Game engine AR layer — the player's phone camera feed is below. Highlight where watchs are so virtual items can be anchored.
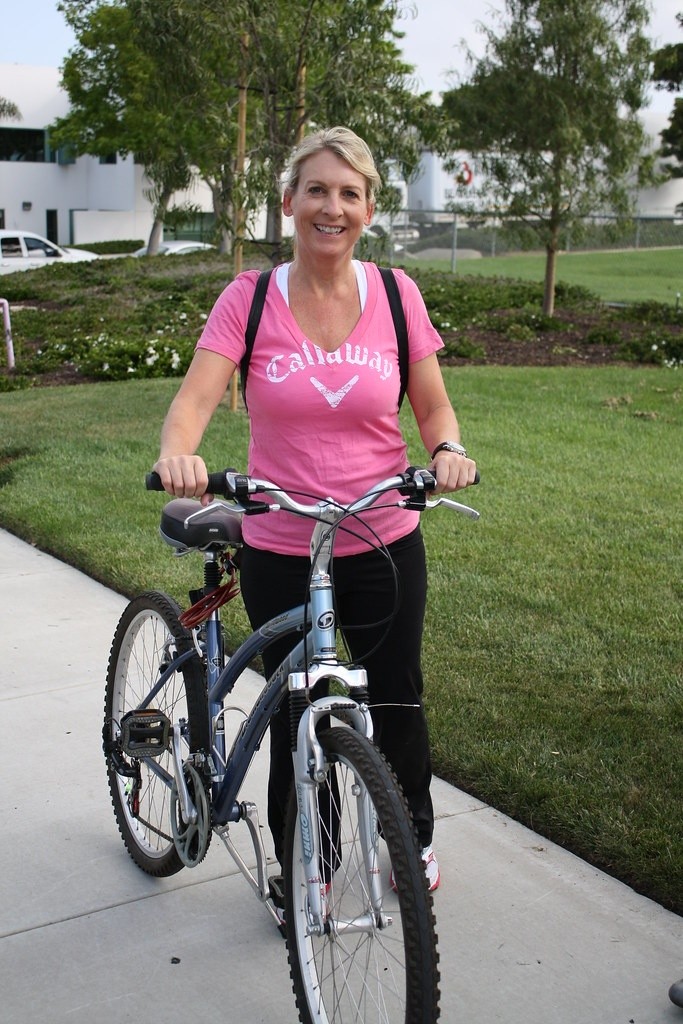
[430,441,468,462]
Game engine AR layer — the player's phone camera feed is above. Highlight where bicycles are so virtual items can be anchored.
[102,469,482,1024]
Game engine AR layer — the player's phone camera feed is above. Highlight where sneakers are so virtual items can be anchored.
[276,880,331,927]
[390,844,440,894]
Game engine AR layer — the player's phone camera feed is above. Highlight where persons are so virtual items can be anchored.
[151,125,476,925]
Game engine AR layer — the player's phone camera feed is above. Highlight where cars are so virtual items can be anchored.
[132,240,217,257]
[0,229,102,276]
